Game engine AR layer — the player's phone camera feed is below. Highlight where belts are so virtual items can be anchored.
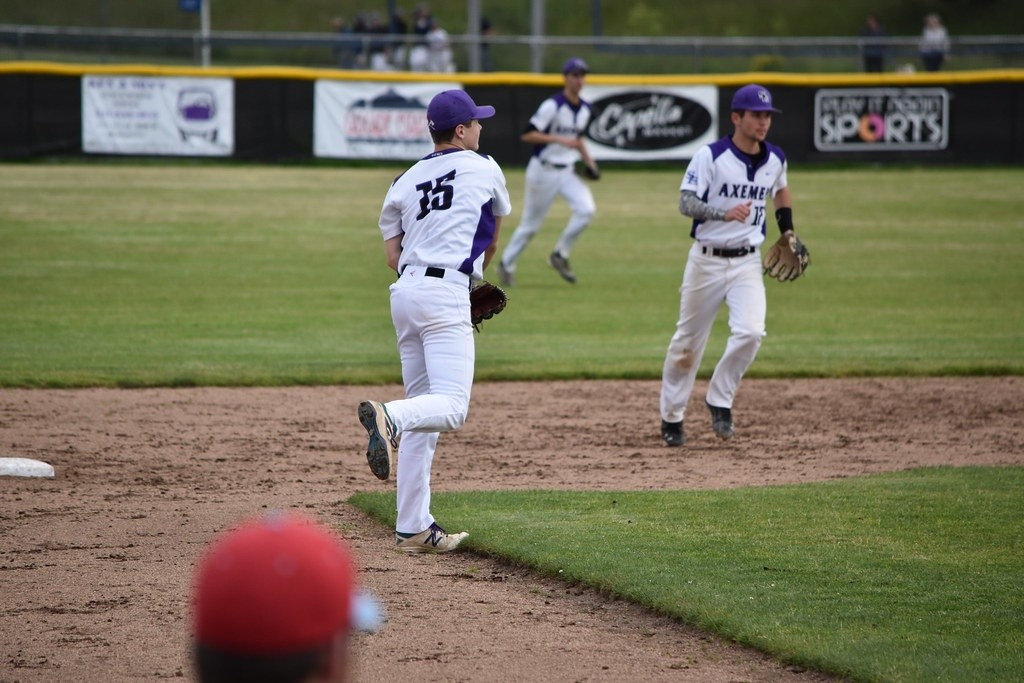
[402,263,445,278]
[704,244,754,258]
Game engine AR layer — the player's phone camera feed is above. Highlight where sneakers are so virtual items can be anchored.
[548,251,575,283]
[357,397,397,479]
[706,398,733,441]
[395,525,470,554]
[661,418,684,446]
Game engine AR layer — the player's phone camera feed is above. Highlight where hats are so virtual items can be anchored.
[564,60,589,75]
[196,522,379,654]
[733,84,780,113]
[426,89,495,132]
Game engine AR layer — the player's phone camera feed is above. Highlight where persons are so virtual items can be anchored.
[658,82,809,448]
[357,89,514,552]
[499,57,602,289]
[858,15,887,74]
[187,511,385,683]
[333,0,493,71]
[918,13,952,74]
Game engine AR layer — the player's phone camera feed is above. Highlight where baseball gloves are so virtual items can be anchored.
[469,279,510,333]
[764,229,813,284]
